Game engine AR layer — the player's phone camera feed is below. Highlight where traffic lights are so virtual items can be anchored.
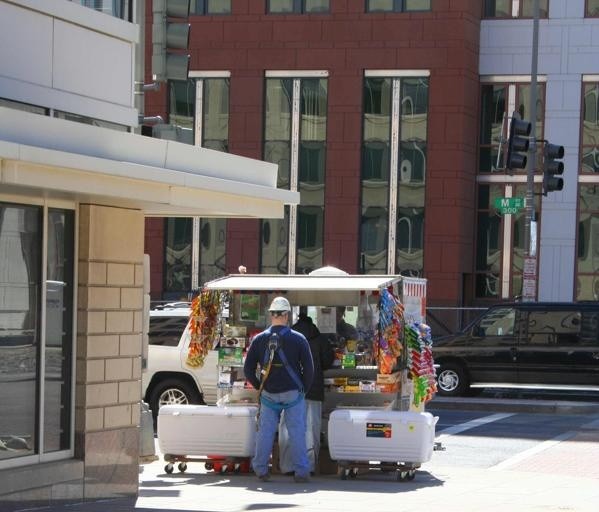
[507,119,532,169]
[543,140,565,192]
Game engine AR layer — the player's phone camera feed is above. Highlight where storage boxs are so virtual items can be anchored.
[157,403,258,459]
[326,407,439,466]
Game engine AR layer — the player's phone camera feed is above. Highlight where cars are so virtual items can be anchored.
[431,301,599,397]
[141,301,230,421]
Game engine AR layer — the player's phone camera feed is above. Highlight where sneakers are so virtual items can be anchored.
[287,471,314,483]
[258,473,271,482]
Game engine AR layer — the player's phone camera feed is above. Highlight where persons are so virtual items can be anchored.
[244,296,335,482]
[325,306,357,340]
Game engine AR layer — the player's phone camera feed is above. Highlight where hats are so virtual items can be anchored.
[336,305,346,318]
[268,297,292,312]
